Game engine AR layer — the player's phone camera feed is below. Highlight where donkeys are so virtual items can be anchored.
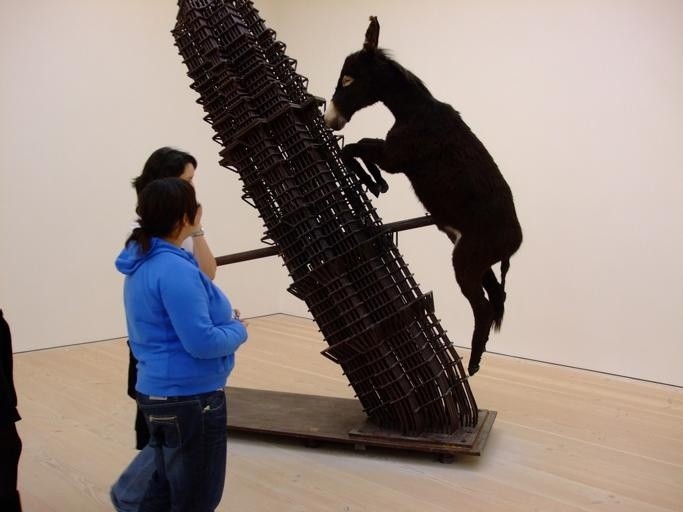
[323,16,522,375]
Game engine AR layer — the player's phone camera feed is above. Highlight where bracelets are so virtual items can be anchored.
[192,229,204,237]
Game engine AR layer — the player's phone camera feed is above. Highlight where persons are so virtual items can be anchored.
[0,310,22,512]
[110,177,249,512]
[128,147,216,451]
